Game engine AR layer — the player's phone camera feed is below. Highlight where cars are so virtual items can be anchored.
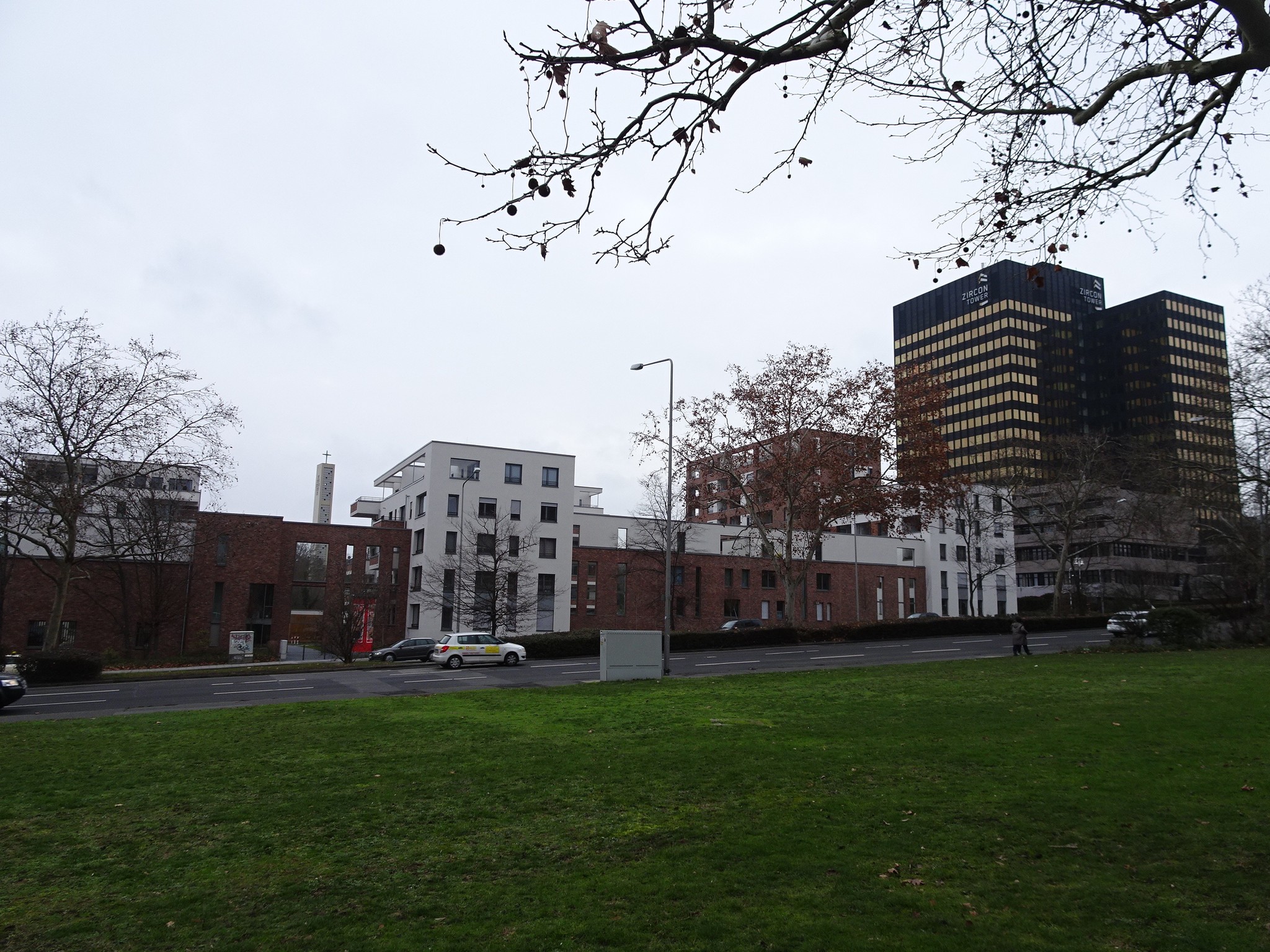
[906,612,941,618]
[368,638,439,663]
[1106,611,1172,638]
[0,651,27,708]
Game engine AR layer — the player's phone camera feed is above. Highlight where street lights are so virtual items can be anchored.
[842,442,860,623]
[631,358,673,675]
[1190,417,1267,611]
[1094,498,1127,613]
[456,468,481,632]
[1069,567,1074,610]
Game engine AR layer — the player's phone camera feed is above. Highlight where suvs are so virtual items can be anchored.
[714,618,764,634]
[430,632,527,669]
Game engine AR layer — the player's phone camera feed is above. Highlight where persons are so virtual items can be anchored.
[1010,615,1033,656]
[1018,617,1026,655]
